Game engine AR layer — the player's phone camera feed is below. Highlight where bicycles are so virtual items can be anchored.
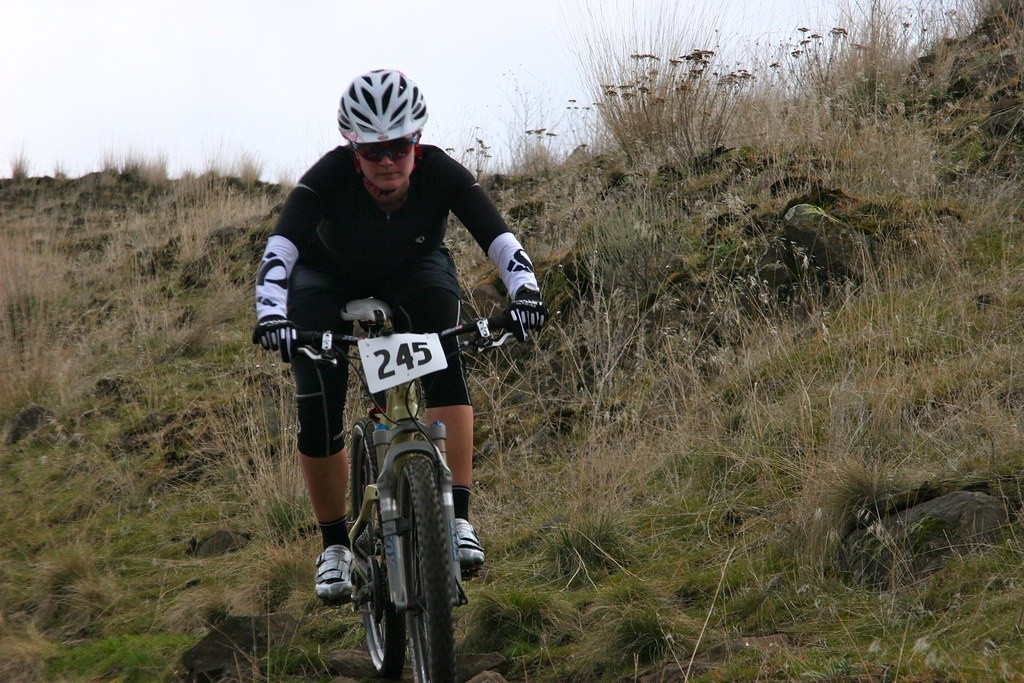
[250,294,527,683]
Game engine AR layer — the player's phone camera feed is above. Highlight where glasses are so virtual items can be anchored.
[348,132,422,162]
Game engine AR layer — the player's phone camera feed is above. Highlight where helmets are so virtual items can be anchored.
[337,70,426,144]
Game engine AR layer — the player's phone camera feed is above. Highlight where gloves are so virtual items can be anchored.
[250,315,298,352]
[505,284,549,330]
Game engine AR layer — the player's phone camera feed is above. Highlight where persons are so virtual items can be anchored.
[252,70,550,605]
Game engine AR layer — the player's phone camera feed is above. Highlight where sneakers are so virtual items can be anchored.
[315,544,355,596]
[452,518,484,564]
